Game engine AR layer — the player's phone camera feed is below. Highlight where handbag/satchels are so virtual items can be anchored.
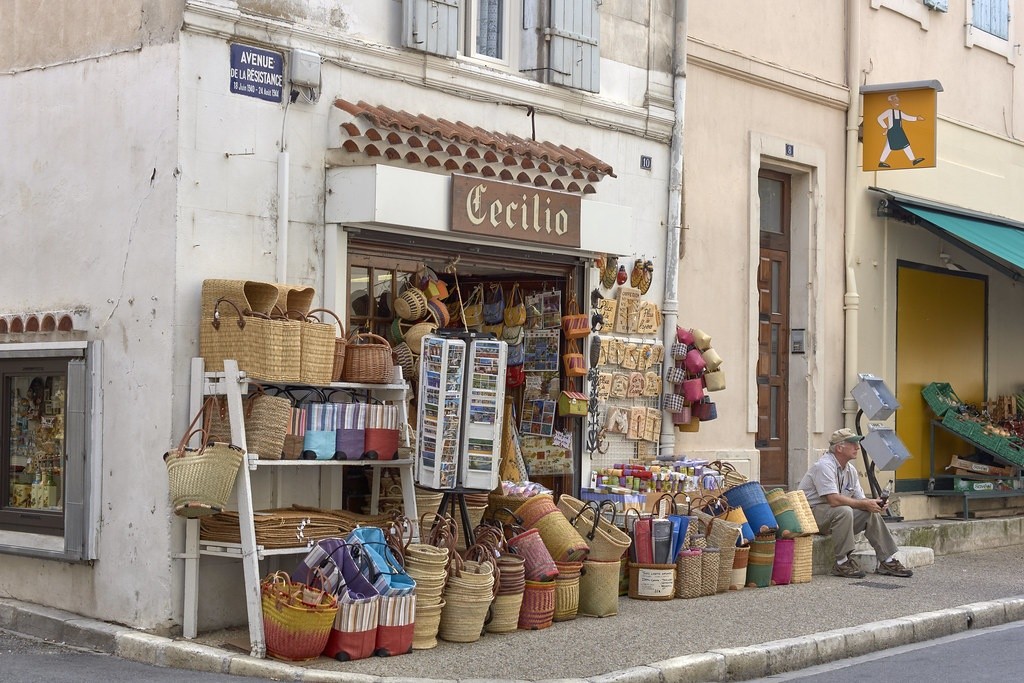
[161,268,819,665]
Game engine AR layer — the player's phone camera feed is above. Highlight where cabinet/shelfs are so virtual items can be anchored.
[180,357,419,659]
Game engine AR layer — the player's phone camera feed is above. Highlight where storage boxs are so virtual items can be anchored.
[919,381,1024,492]
[861,426,911,471]
[850,376,900,421]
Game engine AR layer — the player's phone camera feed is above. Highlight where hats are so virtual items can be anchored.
[829,428,866,445]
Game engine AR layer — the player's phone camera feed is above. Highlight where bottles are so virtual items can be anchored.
[30,467,57,510]
[878,479,894,508]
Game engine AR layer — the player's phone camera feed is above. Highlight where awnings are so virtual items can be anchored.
[869,186,1024,285]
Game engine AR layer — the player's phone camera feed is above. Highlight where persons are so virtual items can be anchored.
[796,427,912,578]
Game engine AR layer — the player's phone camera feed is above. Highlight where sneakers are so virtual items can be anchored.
[877,558,913,577]
[831,557,867,578]
